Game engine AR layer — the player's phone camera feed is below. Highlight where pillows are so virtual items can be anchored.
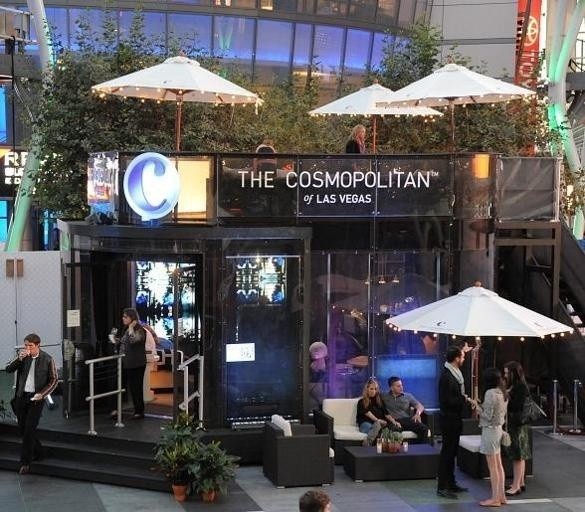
[272,414,292,436]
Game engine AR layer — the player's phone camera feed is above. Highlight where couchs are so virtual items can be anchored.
[311,397,436,463]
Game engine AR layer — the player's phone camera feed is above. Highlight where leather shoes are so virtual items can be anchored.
[506,484,525,496]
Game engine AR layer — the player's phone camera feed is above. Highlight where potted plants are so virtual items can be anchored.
[187,439,239,503]
[154,415,200,503]
[391,431,402,453]
[380,431,391,451]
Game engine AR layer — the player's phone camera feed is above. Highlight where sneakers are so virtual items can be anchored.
[128,414,143,419]
[20,463,29,473]
[480,497,506,507]
[437,485,469,498]
[110,410,118,418]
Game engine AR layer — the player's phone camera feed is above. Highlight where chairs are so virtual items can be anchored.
[262,413,335,487]
[459,412,536,480]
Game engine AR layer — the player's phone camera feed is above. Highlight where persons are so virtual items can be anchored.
[435,345,470,499]
[344,123,367,179]
[467,368,511,507]
[111,308,146,422]
[381,376,429,448]
[5,333,58,475]
[298,489,333,511]
[502,362,533,495]
[356,379,401,449]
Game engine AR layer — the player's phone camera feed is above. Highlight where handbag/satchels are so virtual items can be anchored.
[501,429,511,447]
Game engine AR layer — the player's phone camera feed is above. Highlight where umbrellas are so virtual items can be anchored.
[381,284,576,419]
[305,83,445,156]
[378,63,537,153]
[91,56,261,222]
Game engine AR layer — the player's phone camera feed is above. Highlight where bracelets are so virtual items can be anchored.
[415,411,422,416]
